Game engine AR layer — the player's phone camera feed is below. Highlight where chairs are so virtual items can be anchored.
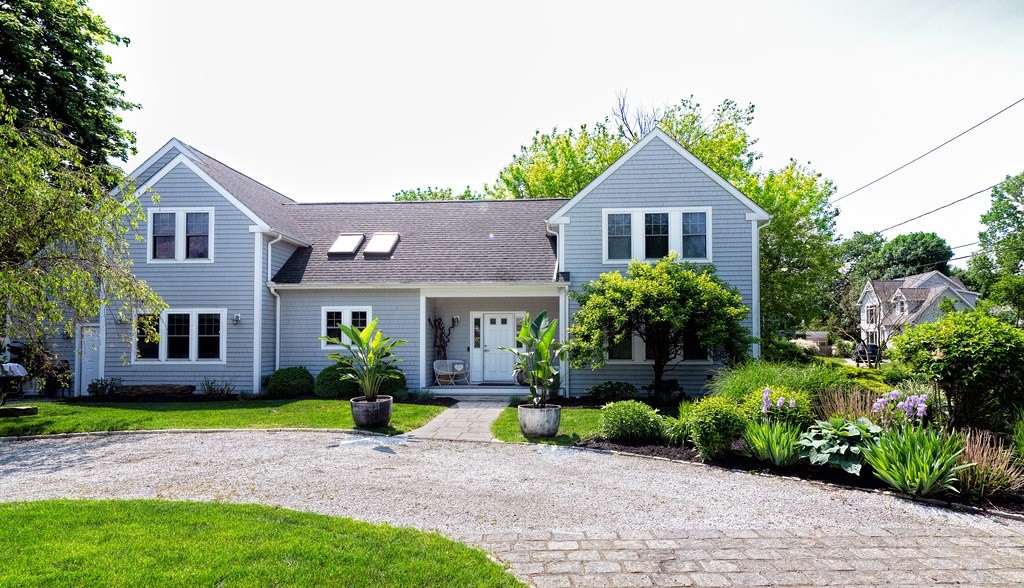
[54,360,71,397]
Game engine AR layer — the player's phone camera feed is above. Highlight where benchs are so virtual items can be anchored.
[431,359,471,387]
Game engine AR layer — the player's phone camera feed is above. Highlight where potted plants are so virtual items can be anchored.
[496,308,570,436]
[317,316,408,430]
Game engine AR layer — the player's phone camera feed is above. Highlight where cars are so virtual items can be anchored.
[852,343,882,364]
